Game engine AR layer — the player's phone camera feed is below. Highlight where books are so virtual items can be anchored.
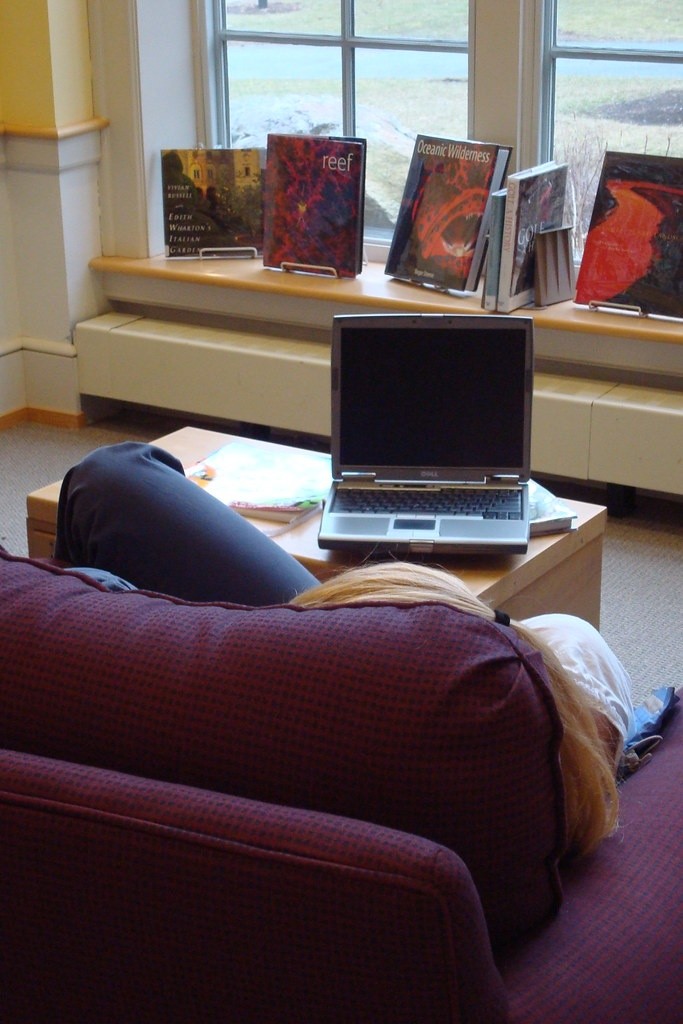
[528,478,579,538]
[572,151,683,320]
[383,133,514,292]
[160,147,263,261]
[226,493,327,525]
[480,161,569,314]
[262,133,367,279]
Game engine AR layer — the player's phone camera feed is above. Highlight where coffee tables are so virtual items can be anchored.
[28,426,609,631]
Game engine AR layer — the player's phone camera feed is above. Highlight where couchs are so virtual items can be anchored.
[1,540,683,1024]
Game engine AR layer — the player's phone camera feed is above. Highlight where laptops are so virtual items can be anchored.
[319,313,536,555]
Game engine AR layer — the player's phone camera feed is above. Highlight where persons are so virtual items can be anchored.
[53,439,631,859]
